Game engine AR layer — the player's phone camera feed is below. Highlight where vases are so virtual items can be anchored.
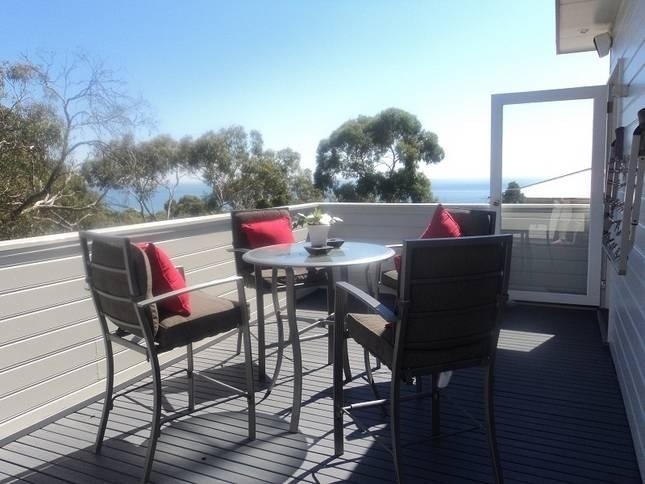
[304,222,330,247]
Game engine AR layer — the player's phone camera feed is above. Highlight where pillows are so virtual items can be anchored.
[394,204,461,273]
[238,214,295,247]
[131,241,191,317]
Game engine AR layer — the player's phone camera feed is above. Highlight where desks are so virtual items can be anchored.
[242,241,398,432]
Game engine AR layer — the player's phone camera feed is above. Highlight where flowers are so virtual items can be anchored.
[289,208,344,228]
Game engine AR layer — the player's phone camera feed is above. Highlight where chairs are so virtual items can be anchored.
[76,224,257,480]
[379,205,500,293]
[230,207,336,382]
[333,232,517,482]
